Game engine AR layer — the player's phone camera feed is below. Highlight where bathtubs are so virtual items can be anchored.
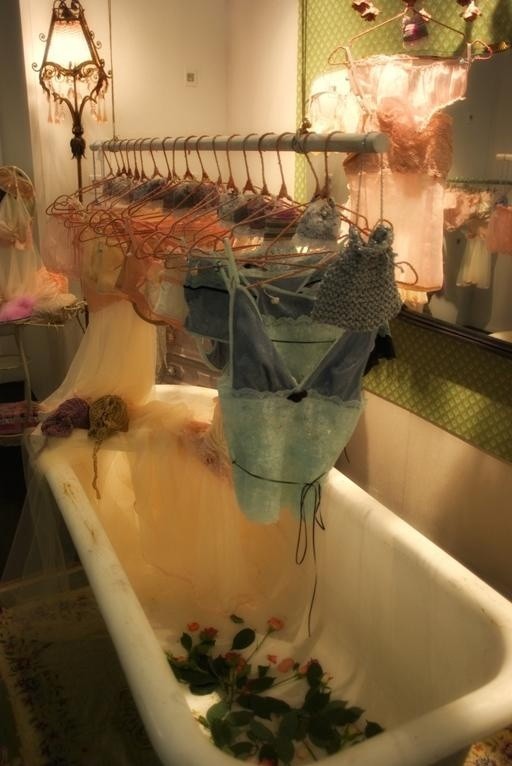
[23,383,512,766]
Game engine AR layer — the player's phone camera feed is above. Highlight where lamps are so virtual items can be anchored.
[31,1,114,329]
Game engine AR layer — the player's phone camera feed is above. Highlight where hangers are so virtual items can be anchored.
[328,0,496,75]
